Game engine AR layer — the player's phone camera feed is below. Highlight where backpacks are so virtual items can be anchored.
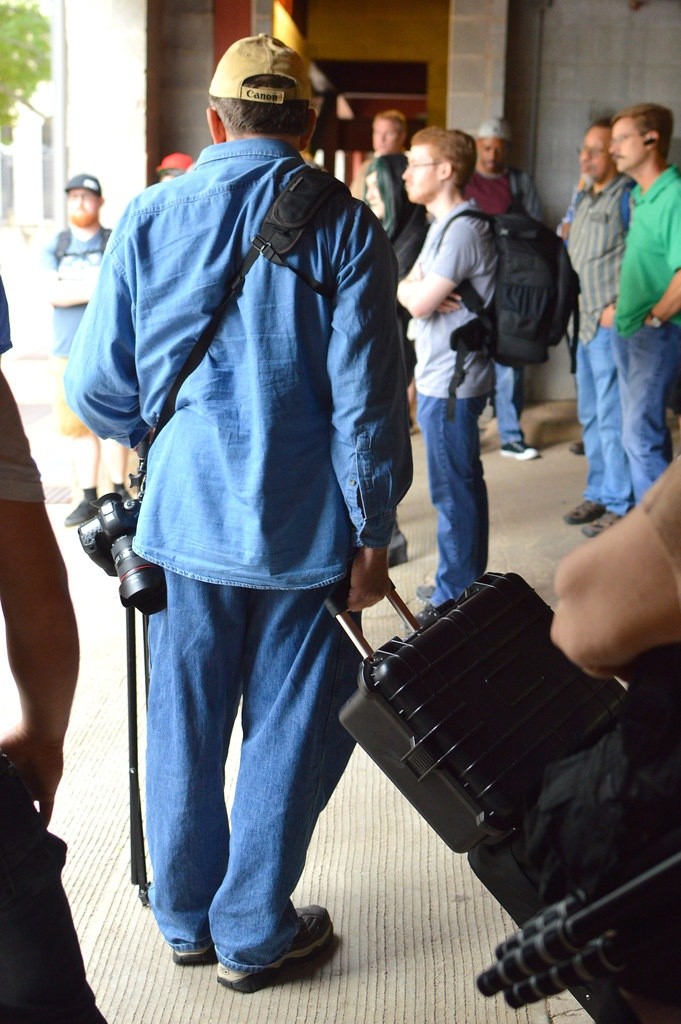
[436,209,579,370]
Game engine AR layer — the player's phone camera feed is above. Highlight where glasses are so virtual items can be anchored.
[577,143,608,154]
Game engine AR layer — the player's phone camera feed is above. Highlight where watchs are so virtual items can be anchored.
[649,313,664,328]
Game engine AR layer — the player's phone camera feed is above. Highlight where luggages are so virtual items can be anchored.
[322,573,651,1024]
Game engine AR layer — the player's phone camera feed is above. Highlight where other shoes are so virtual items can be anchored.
[64,500,98,526]
[415,584,437,604]
[583,512,624,538]
[563,499,605,524]
[500,441,539,460]
[118,490,131,503]
[569,441,585,455]
[402,605,438,632]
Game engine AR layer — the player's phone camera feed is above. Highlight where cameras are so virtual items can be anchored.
[78,496,168,616]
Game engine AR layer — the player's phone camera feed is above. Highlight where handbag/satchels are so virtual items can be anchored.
[524,644,681,1003]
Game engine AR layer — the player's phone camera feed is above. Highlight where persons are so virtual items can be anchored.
[349,109,411,201]
[551,102,681,678]
[563,118,636,538]
[60,32,416,995]
[461,118,547,230]
[45,174,132,527]
[360,151,430,436]
[396,125,497,608]
[0,275,108,1024]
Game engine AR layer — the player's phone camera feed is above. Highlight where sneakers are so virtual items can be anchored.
[216,905,334,993]
[172,943,218,967]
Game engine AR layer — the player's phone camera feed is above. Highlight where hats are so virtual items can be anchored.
[210,33,311,103]
[479,118,514,147]
[65,175,102,197]
[156,153,192,171]
